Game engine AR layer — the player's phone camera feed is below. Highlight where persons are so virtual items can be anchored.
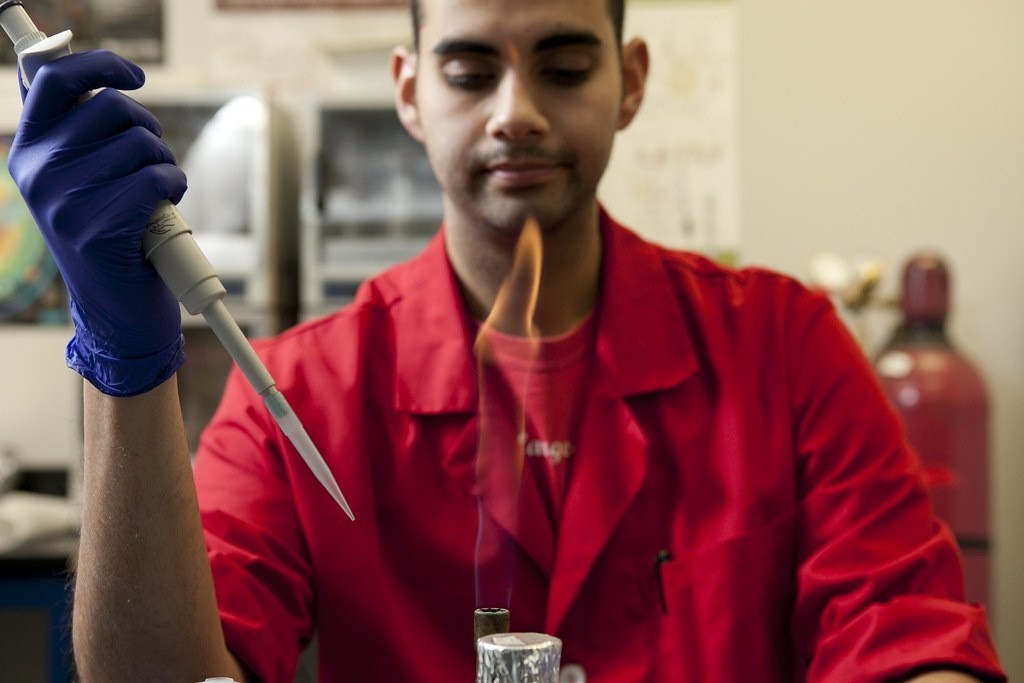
[7,0,1010,683]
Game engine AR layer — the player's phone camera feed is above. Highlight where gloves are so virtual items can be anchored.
[7,47,187,395]
